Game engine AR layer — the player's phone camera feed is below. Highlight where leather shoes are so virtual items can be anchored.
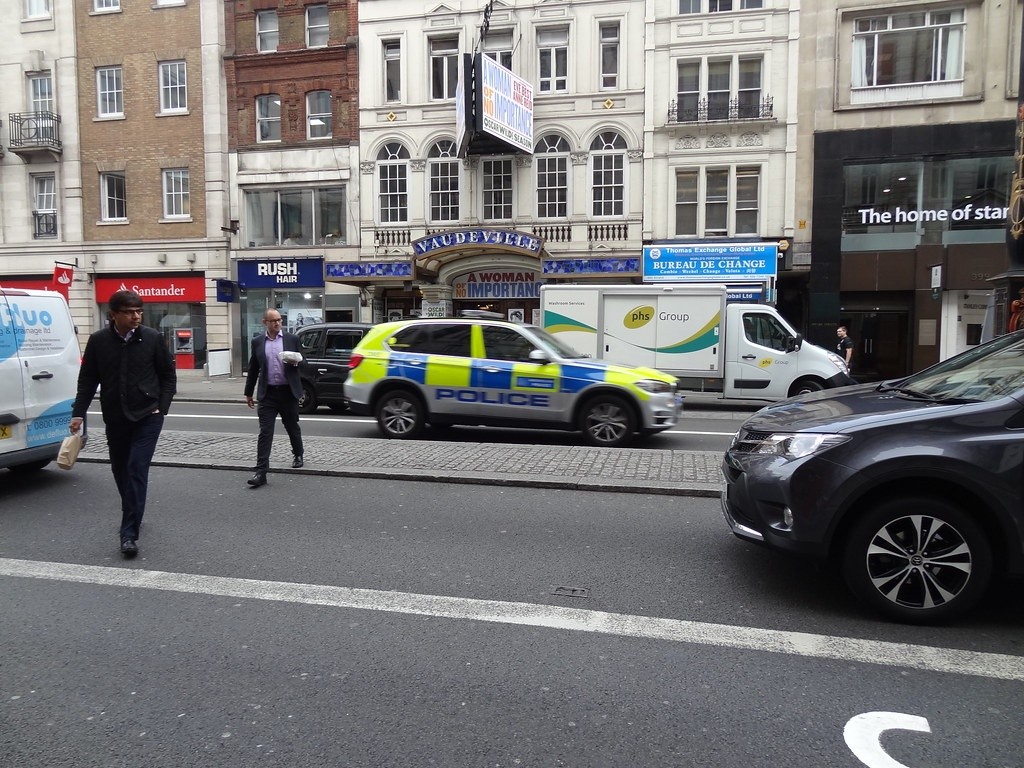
[248,473,267,485]
[292,454,303,468]
[120,535,137,553]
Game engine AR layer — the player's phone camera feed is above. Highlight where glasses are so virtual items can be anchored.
[112,308,144,314]
[265,318,283,323]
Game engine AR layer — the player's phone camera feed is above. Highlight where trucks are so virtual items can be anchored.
[538,283,851,404]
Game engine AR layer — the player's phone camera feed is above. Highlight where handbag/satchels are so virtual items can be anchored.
[57,426,82,471]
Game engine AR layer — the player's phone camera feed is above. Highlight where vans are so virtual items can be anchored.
[0,287,89,473]
[294,322,378,415]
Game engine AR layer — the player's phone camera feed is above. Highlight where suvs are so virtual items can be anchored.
[343,309,685,447]
[723,330,1024,623]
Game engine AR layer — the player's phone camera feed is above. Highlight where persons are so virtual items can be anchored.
[68,290,177,557]
[244,309,307,486]
[835,327,854,369]
